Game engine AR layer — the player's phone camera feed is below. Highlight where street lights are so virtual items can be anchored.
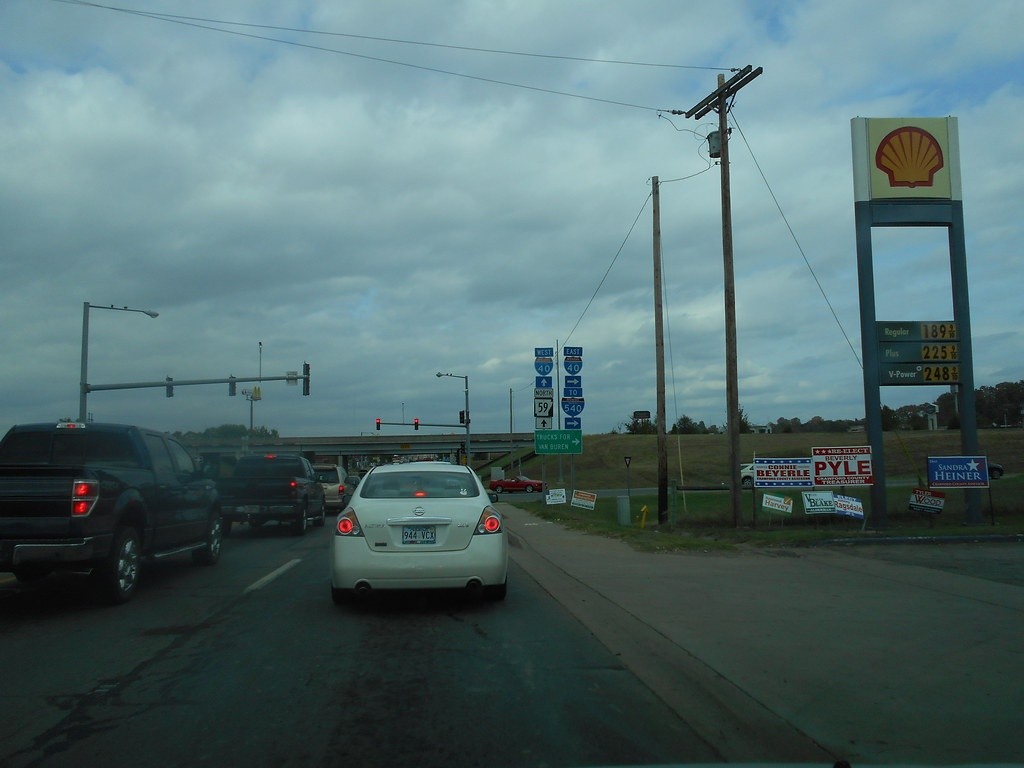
[435,372,471,466]
[242,389,255,431]
[78,301,159,422]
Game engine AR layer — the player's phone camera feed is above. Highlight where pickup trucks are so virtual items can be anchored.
[0,423,225,607]
[216,453,327,538]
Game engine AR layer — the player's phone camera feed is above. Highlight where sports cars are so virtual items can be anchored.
[489,475,548,493]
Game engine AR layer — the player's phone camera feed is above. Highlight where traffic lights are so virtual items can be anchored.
[461,441,466,454]
[375,418,381,430]
[414,418,418,430]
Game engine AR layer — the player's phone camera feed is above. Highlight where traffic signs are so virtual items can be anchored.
[534,429,583,455]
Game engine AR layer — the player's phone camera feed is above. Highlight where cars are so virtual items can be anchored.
[329,462,509,608]
[357,456,434,480]
[740,464,755,489]
[987,461,1004,480]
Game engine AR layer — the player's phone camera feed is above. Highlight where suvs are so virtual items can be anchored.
[313,464,354,513]
